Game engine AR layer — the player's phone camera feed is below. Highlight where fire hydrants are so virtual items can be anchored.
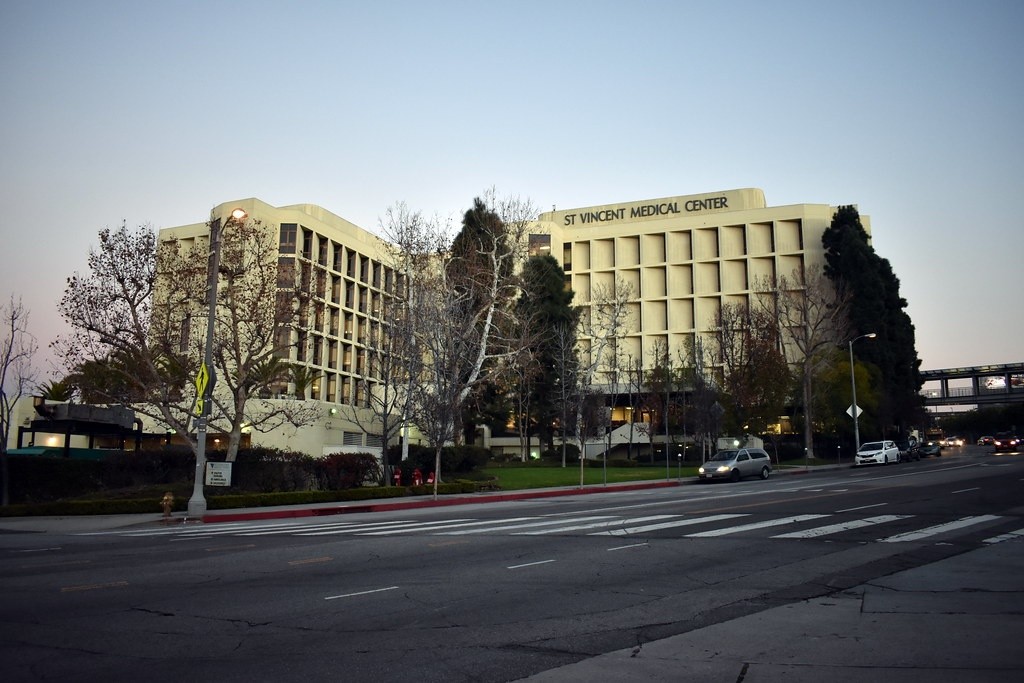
[160,492,175,517]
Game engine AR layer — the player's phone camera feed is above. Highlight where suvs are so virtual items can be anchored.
[900,439,921,462]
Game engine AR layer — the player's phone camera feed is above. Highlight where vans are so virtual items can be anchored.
[855,439,902,467]
[698,447,774,483]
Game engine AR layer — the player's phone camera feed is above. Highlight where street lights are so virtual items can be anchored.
[847,332,877,465]
[184,206,247,521]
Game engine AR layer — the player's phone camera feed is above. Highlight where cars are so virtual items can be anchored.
[994,432,1018,451]
[921,442,942,457]
[976,435,996,445]
[938,435,966,449]
